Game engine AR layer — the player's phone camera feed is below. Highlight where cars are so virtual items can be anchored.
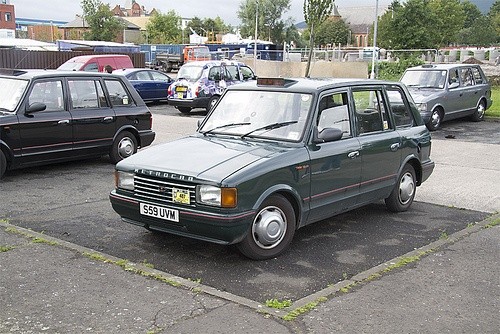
[372,64,493,131]
[0,69,155,178]
[109,78,435,261]
[112,68,175,101]
[167,61,258,114]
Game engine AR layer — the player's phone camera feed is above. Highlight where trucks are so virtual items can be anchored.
[155,46,212,73]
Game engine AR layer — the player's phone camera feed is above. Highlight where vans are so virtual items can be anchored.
[57,54,134,74]
